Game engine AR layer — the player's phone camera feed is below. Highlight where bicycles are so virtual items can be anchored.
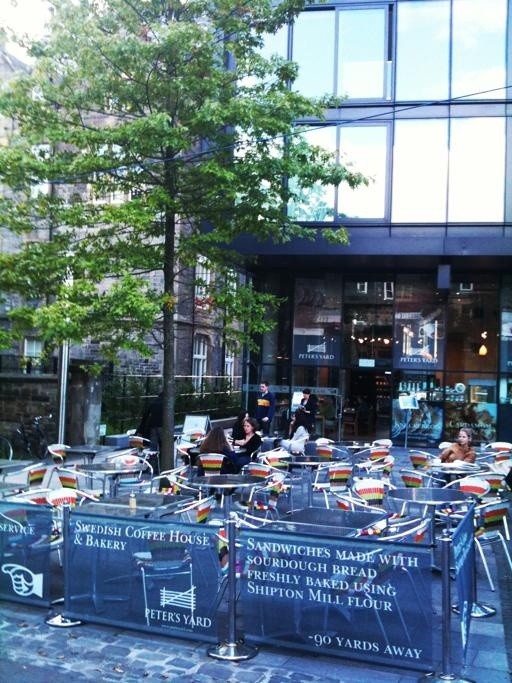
[6,412,54,461]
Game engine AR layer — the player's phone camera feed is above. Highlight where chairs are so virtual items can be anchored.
[2,402,511,661]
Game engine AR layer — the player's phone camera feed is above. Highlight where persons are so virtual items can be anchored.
[297,388,311,408]
[304,395,321,436]
[355,395,370,411]
[195,424,252,500]
[434,425,477,487]
[272,408,311,454]
[255,380,277,435]
[231,417,264,467]
[137,388,164,474]
[319,397,335,426]
[231,410,270,440]
